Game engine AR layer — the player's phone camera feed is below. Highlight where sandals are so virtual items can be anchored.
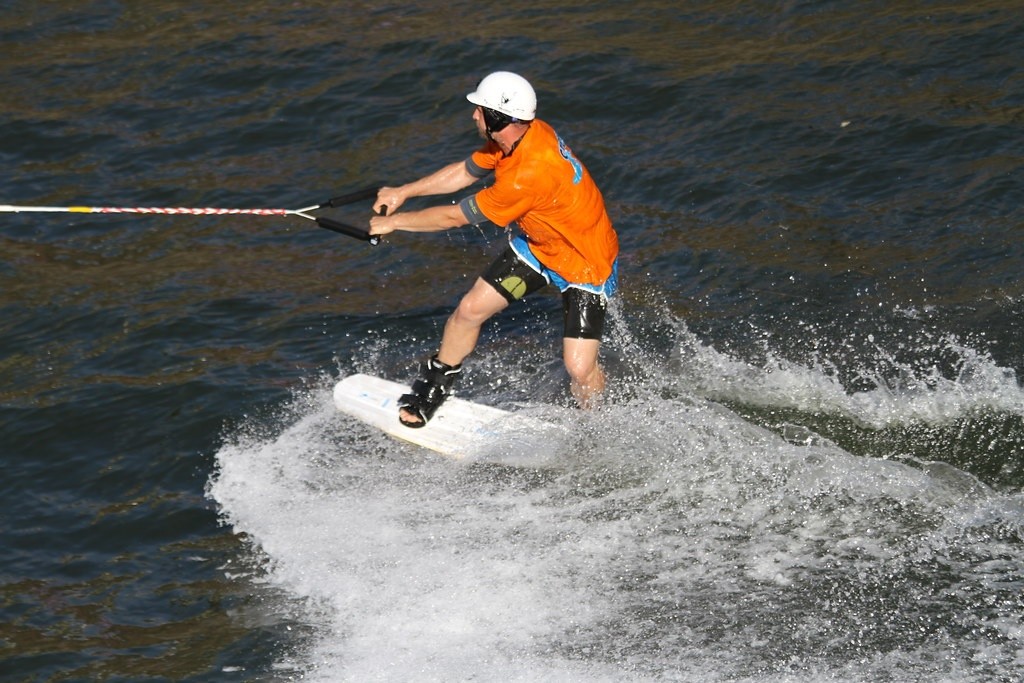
[398,355,461,428]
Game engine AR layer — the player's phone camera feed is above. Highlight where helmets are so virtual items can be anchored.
[466,72,537,120]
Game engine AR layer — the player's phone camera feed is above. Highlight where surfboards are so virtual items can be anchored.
[327,370,570,473]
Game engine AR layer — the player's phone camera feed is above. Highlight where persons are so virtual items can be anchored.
[369,72,619,427]
